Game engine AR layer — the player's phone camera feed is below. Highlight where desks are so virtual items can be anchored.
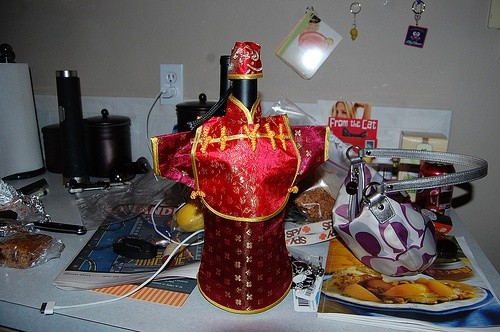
[0,166,499,332]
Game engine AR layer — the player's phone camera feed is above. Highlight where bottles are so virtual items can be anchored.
[214,53,258,117]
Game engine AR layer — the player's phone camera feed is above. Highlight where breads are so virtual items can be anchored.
[295,188,335,223]
[0,229,53,268]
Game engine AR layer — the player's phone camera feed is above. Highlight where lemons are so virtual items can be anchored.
[177,201,205,232]
[343,276,454,303]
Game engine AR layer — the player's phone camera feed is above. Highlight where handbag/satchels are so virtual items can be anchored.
[332,144,489,277]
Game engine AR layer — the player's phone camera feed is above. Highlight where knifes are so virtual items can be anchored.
[0,218,87,235]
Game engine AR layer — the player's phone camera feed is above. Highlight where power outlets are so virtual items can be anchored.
[160,64,184,104]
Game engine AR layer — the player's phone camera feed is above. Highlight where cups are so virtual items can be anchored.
[176,93,217,133]
[82,109,132,176]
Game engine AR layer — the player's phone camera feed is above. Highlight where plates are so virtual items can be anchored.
[320,275,495,320]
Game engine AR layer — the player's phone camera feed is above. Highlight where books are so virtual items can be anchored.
[53,203,206,309]
[316,234,500,332]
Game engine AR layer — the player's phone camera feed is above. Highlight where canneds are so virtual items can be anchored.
[413,161,455,216]
